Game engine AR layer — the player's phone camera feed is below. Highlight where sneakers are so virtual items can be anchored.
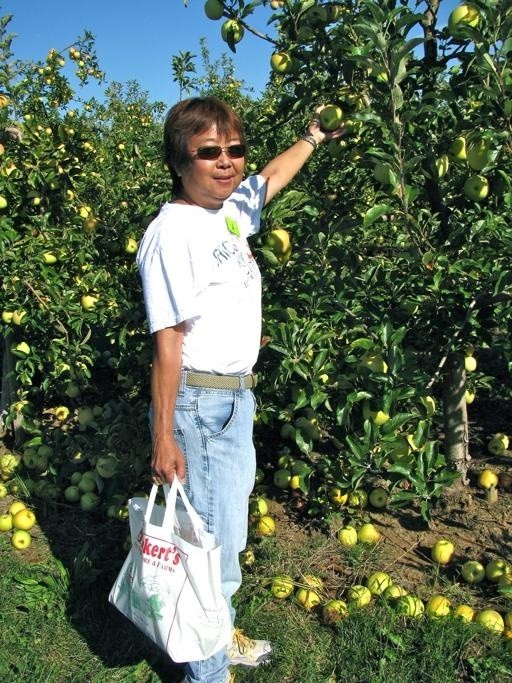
[228,628,277,668]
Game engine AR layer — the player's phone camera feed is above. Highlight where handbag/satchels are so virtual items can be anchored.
[107,475,233,663]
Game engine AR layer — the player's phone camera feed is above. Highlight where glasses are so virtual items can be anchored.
[186,144,245,160]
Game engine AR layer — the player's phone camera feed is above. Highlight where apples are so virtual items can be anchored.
[221,19,244,43]
[0,47,174,550]
[237,1,511,638]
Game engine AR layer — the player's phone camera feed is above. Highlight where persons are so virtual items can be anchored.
[132,96,347,683]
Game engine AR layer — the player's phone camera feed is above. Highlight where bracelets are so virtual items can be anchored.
[301,135,319,149]
